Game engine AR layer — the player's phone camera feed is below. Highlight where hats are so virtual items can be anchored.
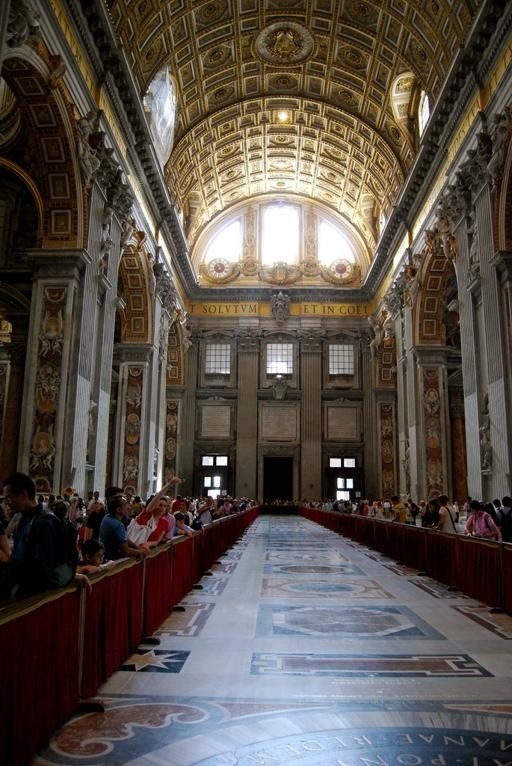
[51,499,70,512]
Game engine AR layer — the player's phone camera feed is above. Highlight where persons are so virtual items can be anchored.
[365,316,382,364]
[265,495,512,542]
[434,209,451,260]
[486,107,510,194]
[78,111,100,174]
[0,476,259,610]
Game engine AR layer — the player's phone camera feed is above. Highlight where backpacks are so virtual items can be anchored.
[28,513,79,588]
[500,509,512,542]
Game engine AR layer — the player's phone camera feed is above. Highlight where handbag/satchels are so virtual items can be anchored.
[126,517,155,546]
